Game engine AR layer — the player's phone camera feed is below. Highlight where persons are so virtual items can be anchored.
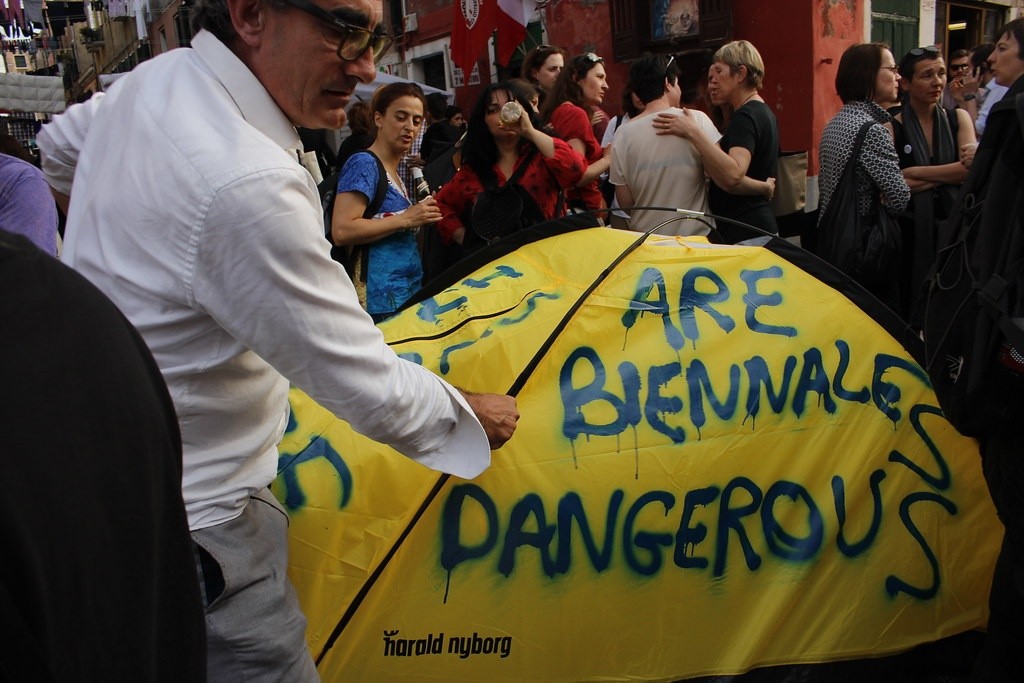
[436,82,588,256]
[510,43,780,241]
[0,134,59,258]
[331,81,443,322]
[1,226,206,681]
[40,0,520,682]
[421,93,467,162]
[335,100,372,178]
[818,17,1024,683]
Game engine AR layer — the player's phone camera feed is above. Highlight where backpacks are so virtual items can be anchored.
[461,146,540,257]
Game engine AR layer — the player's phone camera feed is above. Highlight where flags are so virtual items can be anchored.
[449,0,537,86]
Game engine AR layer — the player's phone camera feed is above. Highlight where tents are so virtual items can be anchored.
[343,72,454,112]
[271,206,1005,683]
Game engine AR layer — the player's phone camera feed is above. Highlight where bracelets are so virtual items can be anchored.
[964,94,976,101]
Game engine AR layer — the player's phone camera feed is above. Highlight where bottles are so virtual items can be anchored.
[497,100,522,126]
[412,166,430,202]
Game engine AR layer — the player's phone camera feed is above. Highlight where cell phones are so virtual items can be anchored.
[973,63,987,78]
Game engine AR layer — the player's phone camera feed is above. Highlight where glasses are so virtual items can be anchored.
[663,53,676,76]
[877,64,899,74]
[949,64,969,71]
[535,44,550,56]
[284,0,394,65]
[907,45,939,60]
[582,53,605,69]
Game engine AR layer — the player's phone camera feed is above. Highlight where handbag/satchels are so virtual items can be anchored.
[816,120,904,299]
[595,114,623,225]
[313,150,388,313]
[422,131,469,196]
[774,149,809,218]
[942,366,1023,439]
